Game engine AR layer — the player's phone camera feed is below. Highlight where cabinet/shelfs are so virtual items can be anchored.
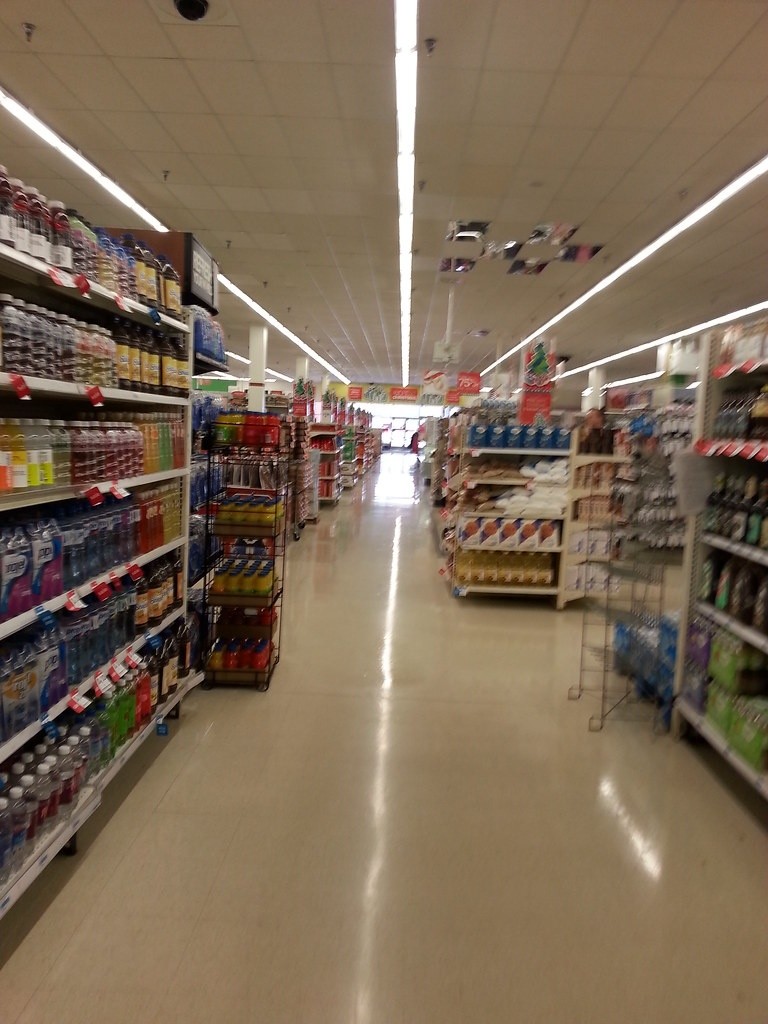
[419,416,675,735]
[188,399,382,690]
[671,330,768,803]
[0,243,204,915]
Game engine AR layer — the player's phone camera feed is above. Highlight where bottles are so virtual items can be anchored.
[606,612,678,719]
[1,167,283,883]
[702,384,767,633]
